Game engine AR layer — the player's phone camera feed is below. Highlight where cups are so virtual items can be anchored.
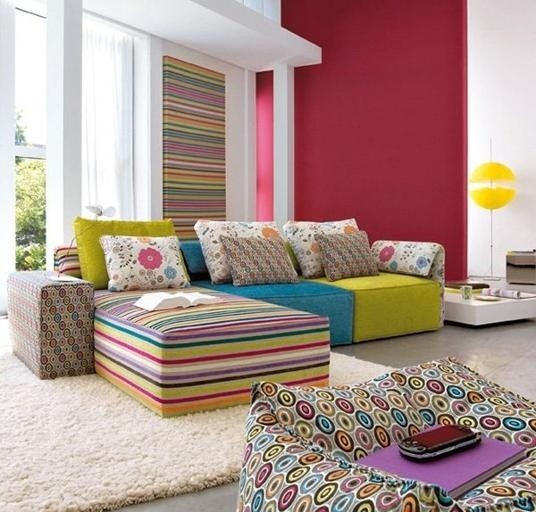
[460,285,473,299]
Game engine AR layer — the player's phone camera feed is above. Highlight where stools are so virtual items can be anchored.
[233,354,536,512]
[505,249,536,285]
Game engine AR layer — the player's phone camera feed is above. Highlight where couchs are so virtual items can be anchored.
[53,238,445,420]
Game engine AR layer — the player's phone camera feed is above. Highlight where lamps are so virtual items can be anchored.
[468,160,520,282]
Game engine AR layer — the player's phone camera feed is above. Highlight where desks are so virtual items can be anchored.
[443,291,536,326]
[6,272,98,379]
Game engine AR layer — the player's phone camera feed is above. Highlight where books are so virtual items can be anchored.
[351,424,527,500]
[446,280,536,302]
[133,290,222,310]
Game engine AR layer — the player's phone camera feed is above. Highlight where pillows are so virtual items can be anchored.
[190,215,284,286]
[218,234,300,289]
[73,213,195,292]
[281,217,360,280]
[316,228,381,282]
[98,232,193,292]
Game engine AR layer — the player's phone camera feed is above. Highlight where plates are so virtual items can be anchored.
[476,295,499,301]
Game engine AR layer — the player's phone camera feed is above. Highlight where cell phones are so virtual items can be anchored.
[398,424,482,463]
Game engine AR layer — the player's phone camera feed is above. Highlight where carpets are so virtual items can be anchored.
[0,347,401,512]
